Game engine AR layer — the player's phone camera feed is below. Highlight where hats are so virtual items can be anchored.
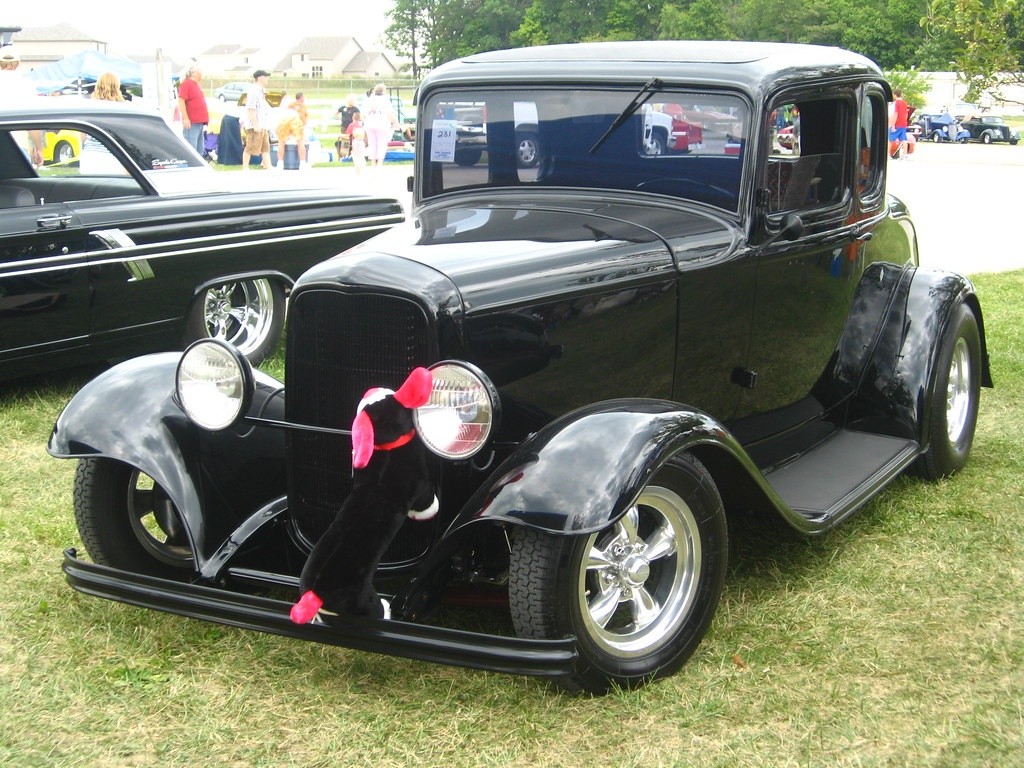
[0,45,20,61]
[366,87,374,97]
[254,70,269,78]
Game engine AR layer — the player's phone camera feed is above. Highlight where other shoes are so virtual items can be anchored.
[888,156,893,160]
[898,154,908,160]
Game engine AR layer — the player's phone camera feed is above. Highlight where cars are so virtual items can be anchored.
[45,40,995,699]
[777,106,916,158]
[0,96,406,369]
[433,103,739,168]
[907,106,923,141]
[216,81,249,102]
[961,114,1021,145]
[912,113,971,144]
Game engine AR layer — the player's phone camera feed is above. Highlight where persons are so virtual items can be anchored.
[240,70,276,171]
[886,88,910,155]
[774,106,788,129]
[0,43,45,170]
[276,93,309,173]
[791,106,800,124]
[333,82,399,172]
[180,58,209,166]
[77,71,128,154]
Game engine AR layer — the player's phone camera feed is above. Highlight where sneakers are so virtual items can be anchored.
[299,163,312,170]
[276,162,285,170]
